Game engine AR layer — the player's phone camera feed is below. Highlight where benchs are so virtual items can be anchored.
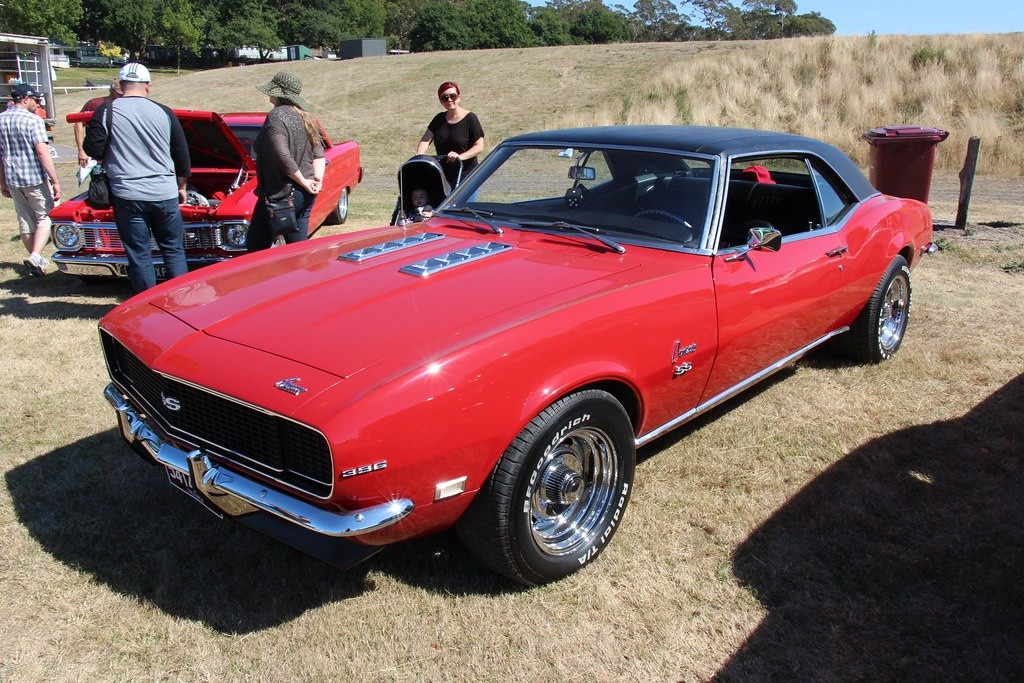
[651,175,821,251]
[84,76,116,90]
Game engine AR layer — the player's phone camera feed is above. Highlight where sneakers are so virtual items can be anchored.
[24,254,46,277]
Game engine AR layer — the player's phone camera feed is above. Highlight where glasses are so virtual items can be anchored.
[439,93,459,103]
[32,97,39,104]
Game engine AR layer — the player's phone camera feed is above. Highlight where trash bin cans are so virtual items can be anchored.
[862,125,950,205]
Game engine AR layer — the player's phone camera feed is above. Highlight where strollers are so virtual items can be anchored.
[390,153,464,226]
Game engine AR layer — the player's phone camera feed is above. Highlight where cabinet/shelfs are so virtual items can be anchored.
[0,56,42,102]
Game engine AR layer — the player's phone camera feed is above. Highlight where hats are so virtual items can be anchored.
[255,70,308,112]
[110,79,123,94]
[118,63,151,83]
[11,84,40,100]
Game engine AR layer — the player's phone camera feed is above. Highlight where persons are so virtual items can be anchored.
[0,84,61,278]
[85,62,192,298]
[74,78,124,187]
[245,70,326,253]
[415,81,485,203]
[2,92,61,213]
[398,186,436,227]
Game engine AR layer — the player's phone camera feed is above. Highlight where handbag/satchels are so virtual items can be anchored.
[265,200,302,237]
[88,172,111,209]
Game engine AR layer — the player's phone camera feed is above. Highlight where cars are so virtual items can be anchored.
[49,109,364,280]
[97,123,935,592]
